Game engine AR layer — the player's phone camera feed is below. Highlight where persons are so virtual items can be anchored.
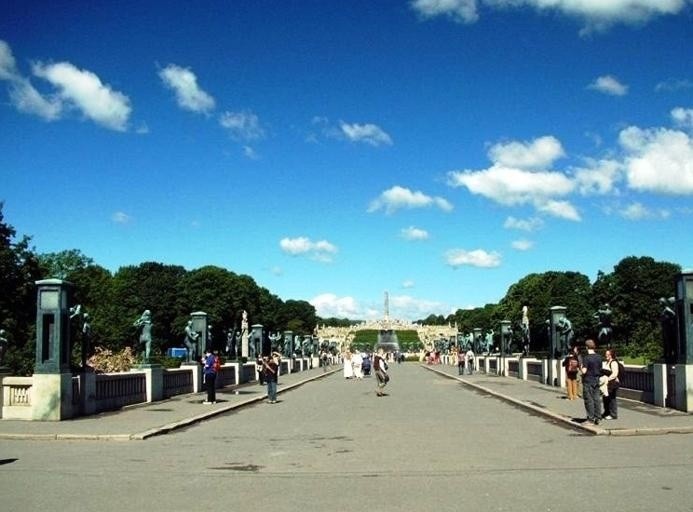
[457,349,465,375]
[464,347,474,375]
[79,312,90,370]
[207,324,319,361]
[573,344,583,399]
[590,302,612,348]
[561,348,583,399]
[256,349,282,386]
[133,309,155,362]
[657,296,676,363]
[578,339,603,426]
[374,347,389,396]
[602,349,621,420]
[199,348,217,404]
[182,319,200,364]
[433,305,576,361]
[241,310,249,334]
[261,355,277,403]
[319,344,458,380]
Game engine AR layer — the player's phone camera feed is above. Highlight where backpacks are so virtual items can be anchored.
[610,360,624,380]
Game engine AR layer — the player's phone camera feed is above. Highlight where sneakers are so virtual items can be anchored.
[583,415,612,426]
